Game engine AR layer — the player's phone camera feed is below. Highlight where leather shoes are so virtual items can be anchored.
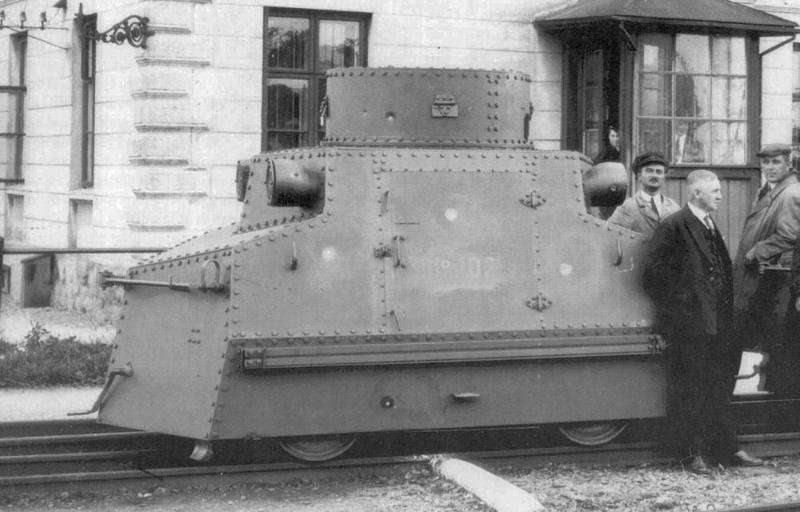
[735,450,764,466]
[686,454,708,474]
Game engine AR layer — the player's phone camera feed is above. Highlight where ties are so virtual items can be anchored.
[649,197,660,217]
[704,215,714,235]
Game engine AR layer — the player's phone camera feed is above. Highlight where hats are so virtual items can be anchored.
[757,144,790,157]
[632,150,667,174]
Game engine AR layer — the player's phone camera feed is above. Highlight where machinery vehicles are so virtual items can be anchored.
[66,67,748,464]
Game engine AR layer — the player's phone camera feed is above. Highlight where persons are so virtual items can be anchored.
[641,170,764,474]
[593,123,621,164]
[734,143,800,390]
[606,151,681,236]
